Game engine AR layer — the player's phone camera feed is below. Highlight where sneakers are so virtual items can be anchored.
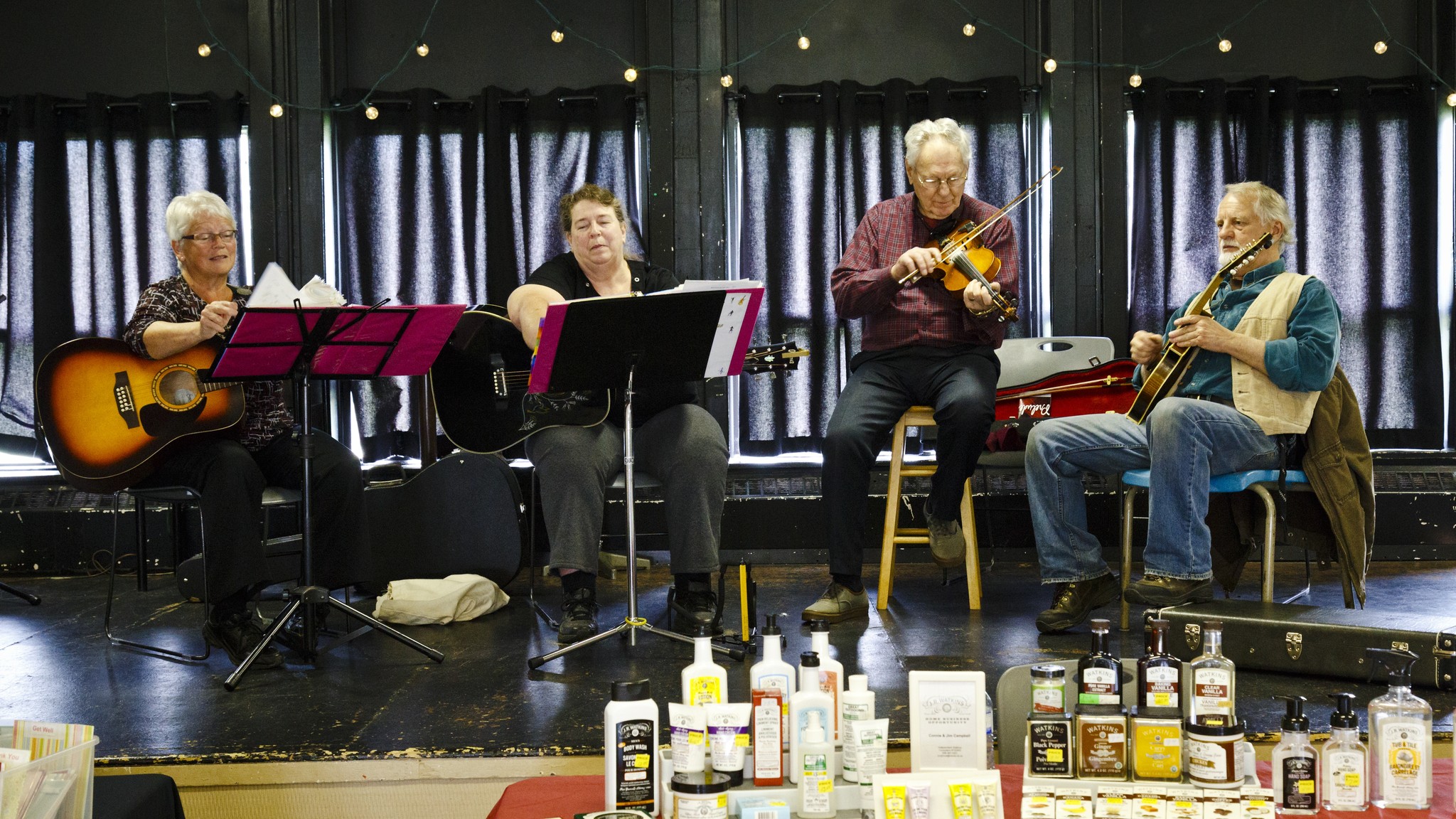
[1035,572,1122,632]
[801,580,869,624]
[557,589,602,643]
[923,497,967,568]
[1123,573,1214,608]
[674,590,723,638]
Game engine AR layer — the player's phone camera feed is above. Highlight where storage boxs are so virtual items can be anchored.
[0,726,100,819]
[1141,596,1456,692]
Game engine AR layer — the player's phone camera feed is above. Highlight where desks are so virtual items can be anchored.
[92,773,186,819]
[486,759,1456,819]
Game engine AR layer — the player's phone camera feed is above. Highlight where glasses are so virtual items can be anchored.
[914,166,968,189]
[182,229,239,243]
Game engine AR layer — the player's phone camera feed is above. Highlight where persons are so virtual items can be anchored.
[1025,180,1343,634]
[507,183,730,645]
[122,189,362,672]
[799,118,1018,630]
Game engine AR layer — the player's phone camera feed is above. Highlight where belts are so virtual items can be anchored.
[1172,394,1236,408]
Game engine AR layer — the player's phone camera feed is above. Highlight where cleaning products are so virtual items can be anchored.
[1364,644,1436,811]
[1271,692,1322,815]
[1321,691,1371,815]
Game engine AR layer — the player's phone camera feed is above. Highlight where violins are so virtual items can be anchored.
[923,220,1020,325]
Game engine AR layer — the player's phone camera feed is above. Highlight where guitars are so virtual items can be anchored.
[429,303,810,455]
[1125,232,1273,424]
[36,336,249,479]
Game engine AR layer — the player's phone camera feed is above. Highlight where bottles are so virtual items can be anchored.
[1136,619,1183,715]
[985,692,994,770]
[1077,618,1122,712]
[1190,621,1236,726]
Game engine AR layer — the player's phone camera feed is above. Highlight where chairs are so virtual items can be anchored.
[103,481,305,663]
[1119,361,1376,632]
[995,656,1236,766]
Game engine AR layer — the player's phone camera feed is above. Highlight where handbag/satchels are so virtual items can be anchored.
[371,574,510,625]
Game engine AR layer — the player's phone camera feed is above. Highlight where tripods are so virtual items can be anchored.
[197,297,468,691]
[522,286,766,669]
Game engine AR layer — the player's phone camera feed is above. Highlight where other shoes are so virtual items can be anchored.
[281,592,332,651]
[200,608,286,668]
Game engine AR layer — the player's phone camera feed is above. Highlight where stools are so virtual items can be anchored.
[875,405,984,611]
[537,467,662,581]
